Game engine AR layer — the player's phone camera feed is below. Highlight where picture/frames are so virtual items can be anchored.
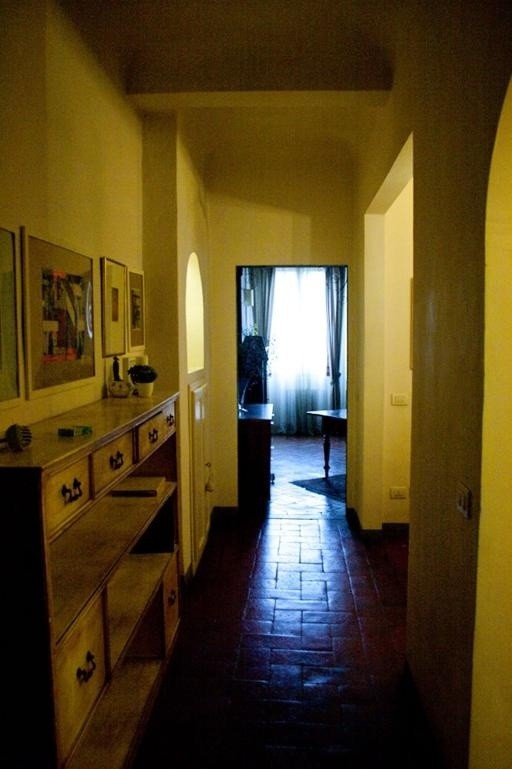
[0,218,147,414]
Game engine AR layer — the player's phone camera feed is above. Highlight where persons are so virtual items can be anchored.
[35,266,87,364]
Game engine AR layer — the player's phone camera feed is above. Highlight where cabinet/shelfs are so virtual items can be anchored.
[1,390,184,768]
[237,401,276,507]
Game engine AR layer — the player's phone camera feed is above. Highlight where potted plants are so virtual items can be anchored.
[238,327,272,404]
[125,362,161,397]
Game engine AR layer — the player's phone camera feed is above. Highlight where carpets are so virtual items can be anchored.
[287,473,347,505]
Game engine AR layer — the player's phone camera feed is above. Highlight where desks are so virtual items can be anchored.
[304,408,348,481]
